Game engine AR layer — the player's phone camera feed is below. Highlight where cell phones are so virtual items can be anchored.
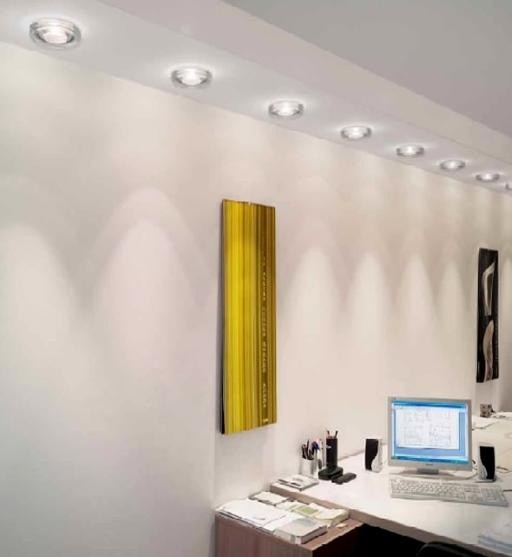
[335,472,356,484]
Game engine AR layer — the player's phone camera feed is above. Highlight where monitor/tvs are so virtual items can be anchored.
[386,396,472,479]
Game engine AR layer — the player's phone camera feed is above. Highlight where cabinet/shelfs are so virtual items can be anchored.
[213,488,366,557]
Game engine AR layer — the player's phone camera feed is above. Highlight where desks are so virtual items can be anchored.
[270,403,512,557]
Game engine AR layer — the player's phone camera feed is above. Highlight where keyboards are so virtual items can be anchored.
[389,476,509,507]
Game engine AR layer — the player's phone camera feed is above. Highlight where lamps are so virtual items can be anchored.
[395,143,425,160]
[505,182,512,190]
[268,98,306,123]
[338,122,372,144]
[474,168,500,186]
[27,15,87,55]
[167,62,212,92]
[439,157,466,174]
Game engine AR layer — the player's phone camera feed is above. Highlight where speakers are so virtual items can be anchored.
[475,442,496,483]
[365,438,382,472]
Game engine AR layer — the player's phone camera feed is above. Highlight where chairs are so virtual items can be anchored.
[415,540,486,557]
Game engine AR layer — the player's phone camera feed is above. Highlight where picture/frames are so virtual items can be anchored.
[474,246,503,384]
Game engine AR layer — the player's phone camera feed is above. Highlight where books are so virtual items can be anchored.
[215,471,350,543]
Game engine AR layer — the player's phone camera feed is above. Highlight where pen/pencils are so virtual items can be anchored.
[327,430,338,439]
[301,439,319,460]
